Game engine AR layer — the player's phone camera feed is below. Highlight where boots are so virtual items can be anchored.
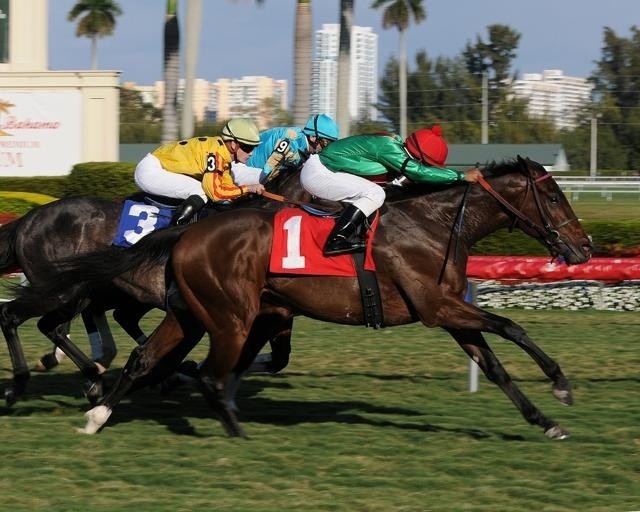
[323,204,366,255]
[173,204,194,226]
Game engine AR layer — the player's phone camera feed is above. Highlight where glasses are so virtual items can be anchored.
[239,143,254,153]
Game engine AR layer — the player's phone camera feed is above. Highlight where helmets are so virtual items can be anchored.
[406,126,448,168]
[303,114,338,141]
[222,119,260,145]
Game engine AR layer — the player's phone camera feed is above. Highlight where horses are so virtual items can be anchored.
[0,151,596,443]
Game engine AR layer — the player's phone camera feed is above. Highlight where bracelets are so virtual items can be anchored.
[238,185,244,197]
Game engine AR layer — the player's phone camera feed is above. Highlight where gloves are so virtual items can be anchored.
[249,184,265,195]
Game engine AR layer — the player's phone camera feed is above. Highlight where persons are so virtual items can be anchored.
[230,112,342,186]
[300,125,486,257]
[133,115,266,225]
